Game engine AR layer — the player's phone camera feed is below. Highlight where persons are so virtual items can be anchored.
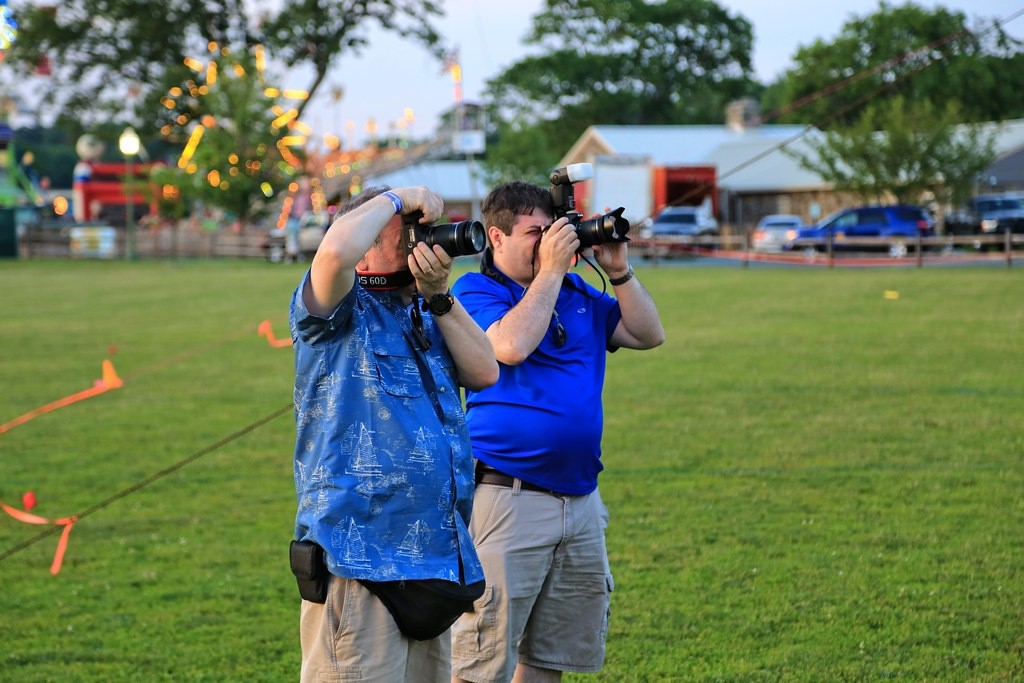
[289,181,501,683]
[449,181,667,683]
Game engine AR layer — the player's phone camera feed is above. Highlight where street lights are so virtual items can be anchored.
[119,127,140,261]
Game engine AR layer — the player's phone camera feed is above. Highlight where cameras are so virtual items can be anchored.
[402,210,486,267]
[549,163,631,255]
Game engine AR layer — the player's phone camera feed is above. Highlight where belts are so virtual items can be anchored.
[474,467,564,500]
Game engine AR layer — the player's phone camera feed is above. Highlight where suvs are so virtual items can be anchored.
[782,204,936,253]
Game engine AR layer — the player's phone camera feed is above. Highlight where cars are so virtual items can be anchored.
[639,205,718,261]
[752,216,803,255]
[945,194,1024,253]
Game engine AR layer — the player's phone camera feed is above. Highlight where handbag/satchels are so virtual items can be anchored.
[357,579,488,645]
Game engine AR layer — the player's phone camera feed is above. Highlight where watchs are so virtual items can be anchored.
[421,287,455,317]
[608,264,635,286]
